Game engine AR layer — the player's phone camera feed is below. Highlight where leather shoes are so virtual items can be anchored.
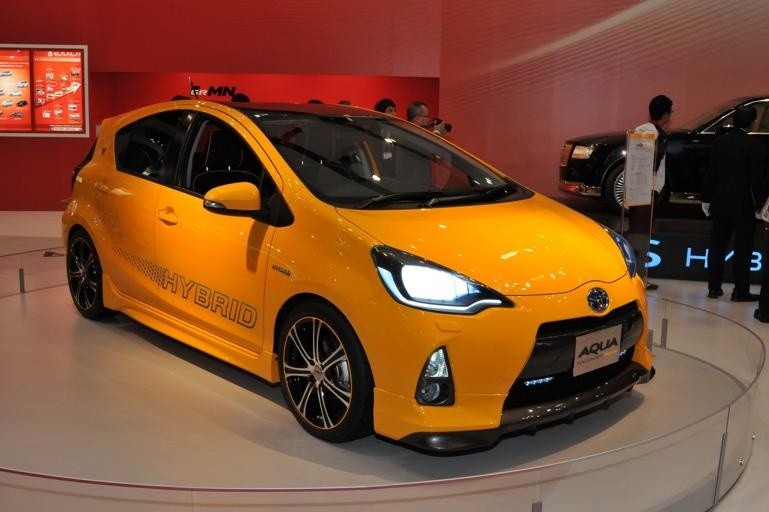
[731,288,760,302]
[754,308,769,324]
[707,289,724,300]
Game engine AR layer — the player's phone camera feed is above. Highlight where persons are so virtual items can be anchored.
[170,94,452,187]
[624,95,674,290]
[700,106,769,302]
[754,219,769,323]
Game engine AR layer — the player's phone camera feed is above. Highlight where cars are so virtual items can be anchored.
[59,76,658,454]
[0,70,29,117]
[557,95,769,216]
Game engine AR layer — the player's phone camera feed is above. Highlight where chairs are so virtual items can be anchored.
[192,130,260,197]
[293,122,354,193]
